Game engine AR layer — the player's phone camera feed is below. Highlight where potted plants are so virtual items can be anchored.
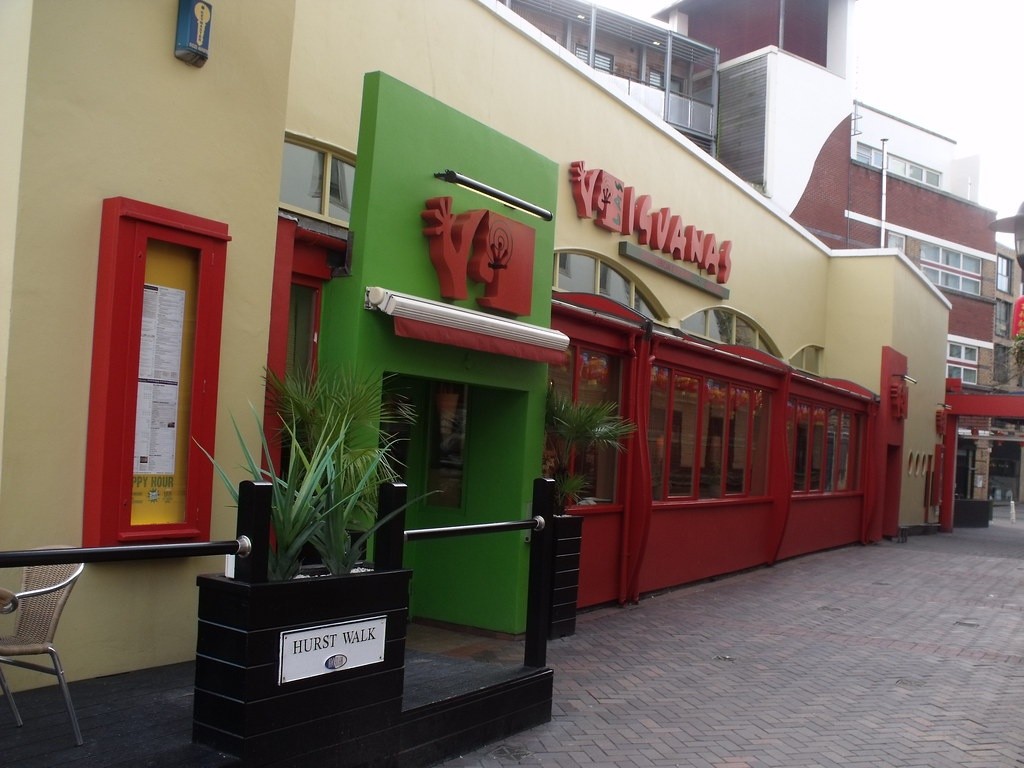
[545,389,637,643]
[191,360,443,759]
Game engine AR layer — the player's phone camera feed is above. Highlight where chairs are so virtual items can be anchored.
[0,545,85,746]
